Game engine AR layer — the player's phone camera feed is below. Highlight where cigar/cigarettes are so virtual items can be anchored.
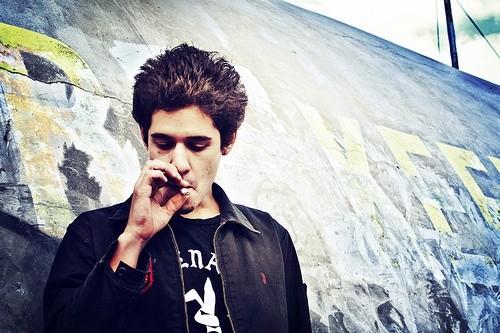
[178,186,190,195]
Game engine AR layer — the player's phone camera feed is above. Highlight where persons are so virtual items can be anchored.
[43,41,312,333]
